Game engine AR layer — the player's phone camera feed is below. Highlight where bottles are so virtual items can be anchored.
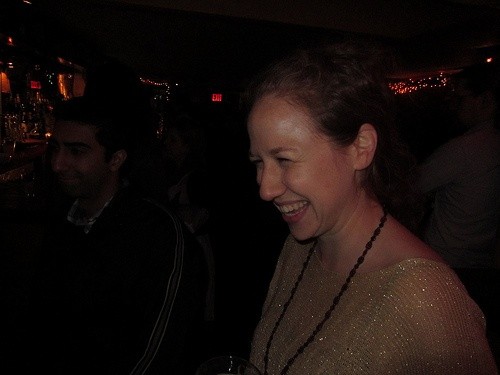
[18,101,44,140]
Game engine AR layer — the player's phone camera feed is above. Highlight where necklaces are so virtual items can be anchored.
[262,200,389,375]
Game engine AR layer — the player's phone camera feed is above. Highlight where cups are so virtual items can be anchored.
[3,113,22,140]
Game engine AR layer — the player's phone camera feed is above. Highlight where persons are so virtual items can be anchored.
[157,121,215,261]
[409,62,500,372]
[1,94,207,375]
[245,43,499,375]
[453,72,467,96]
